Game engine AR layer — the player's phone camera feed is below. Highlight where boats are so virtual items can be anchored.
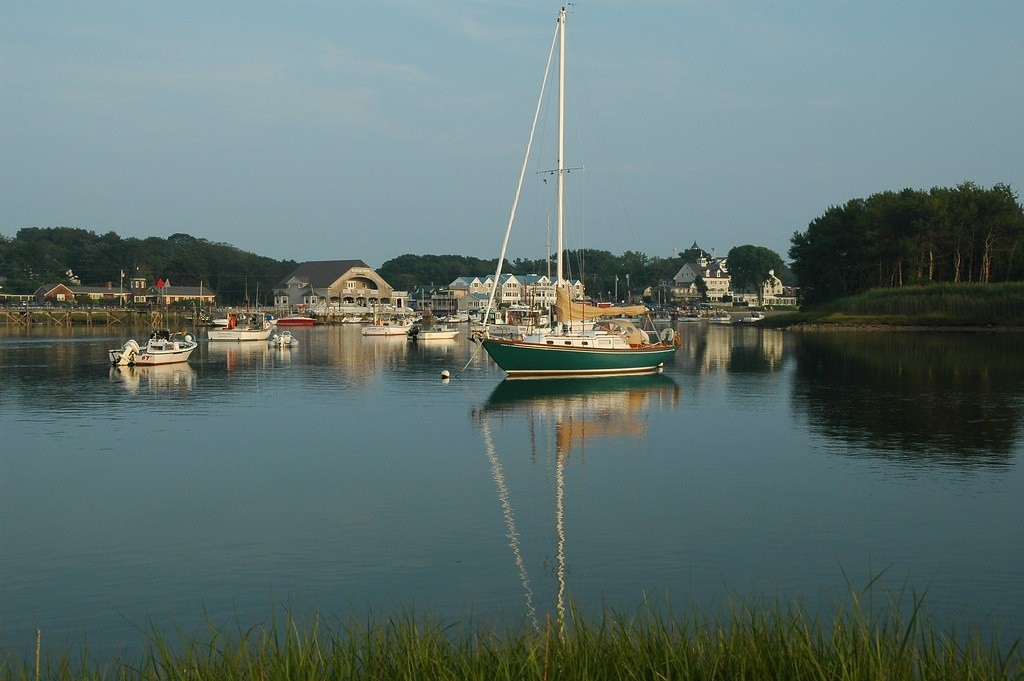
[205,302,469,329]
[611,318,640,322]
[710,310,731,321]
[677,313,702,321]
[653,311,672,322]
[108,363,196,394]
[742,311,765,322]
[205,312,273,340]
[109,330,198,366]
[405,324,461,340]
[361,325,411,336]
[269,330,299,349]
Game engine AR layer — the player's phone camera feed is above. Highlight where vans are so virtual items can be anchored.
[19,301,29,306]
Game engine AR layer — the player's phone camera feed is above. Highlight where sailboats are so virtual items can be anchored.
[470,375,686,650]
[473,280,540,335]
[533,214,557,335]
[452,10,682,373]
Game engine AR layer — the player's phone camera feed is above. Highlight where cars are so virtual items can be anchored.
[30,302,135,309]
[645,303,717,311]
